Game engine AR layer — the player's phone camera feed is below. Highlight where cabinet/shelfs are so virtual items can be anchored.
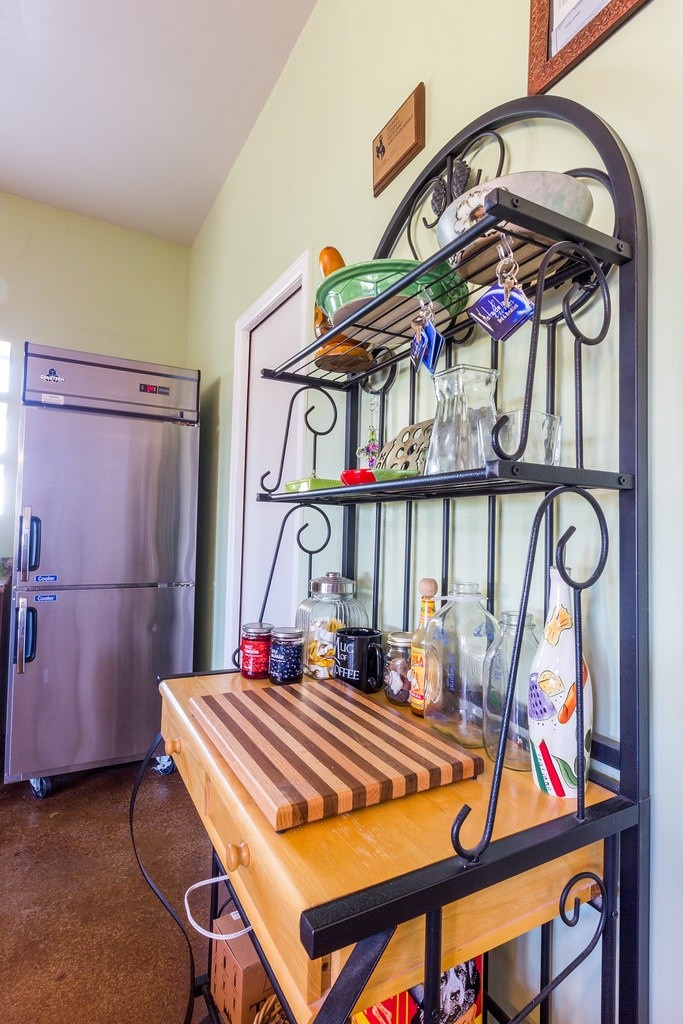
[130,97,649,1024]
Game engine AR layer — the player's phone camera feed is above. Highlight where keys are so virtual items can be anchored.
[501,279,514,306]
[411,316,425,342]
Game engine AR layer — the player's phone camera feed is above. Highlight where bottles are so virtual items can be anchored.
[527,566,593,798]
[424,364,501,475]
[410,579,444,718]
[425,581,501,749]
[483,611,540,772]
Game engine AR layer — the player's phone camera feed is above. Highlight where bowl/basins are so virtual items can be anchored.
[436,170,593,286]
[314,259,469,345]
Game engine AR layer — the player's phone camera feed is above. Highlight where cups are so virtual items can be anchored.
[334,627,384,694]
[477,408,563,470]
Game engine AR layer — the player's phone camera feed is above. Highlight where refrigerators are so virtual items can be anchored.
[0,340,204,800]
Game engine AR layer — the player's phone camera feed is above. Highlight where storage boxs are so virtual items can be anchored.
[211,911,276,1024]
[528,0,650,97]
